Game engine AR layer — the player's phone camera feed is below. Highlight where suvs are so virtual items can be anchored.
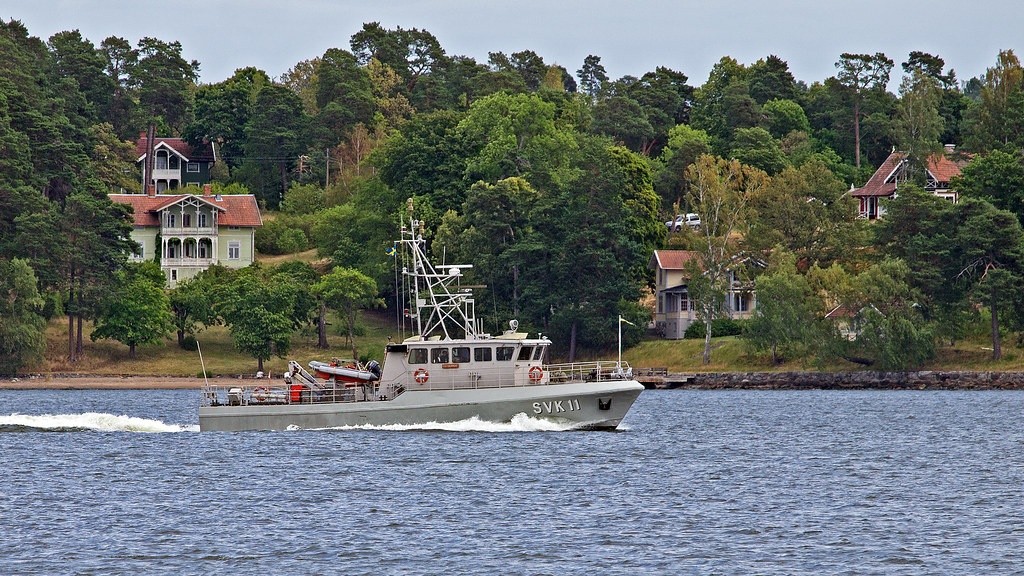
[665,213,701,232]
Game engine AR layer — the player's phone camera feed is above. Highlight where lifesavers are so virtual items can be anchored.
[253,385,268,402]
[414,367,430,384]
[529,366,544,382]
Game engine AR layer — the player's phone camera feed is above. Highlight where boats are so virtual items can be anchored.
[195,197,645,433]
